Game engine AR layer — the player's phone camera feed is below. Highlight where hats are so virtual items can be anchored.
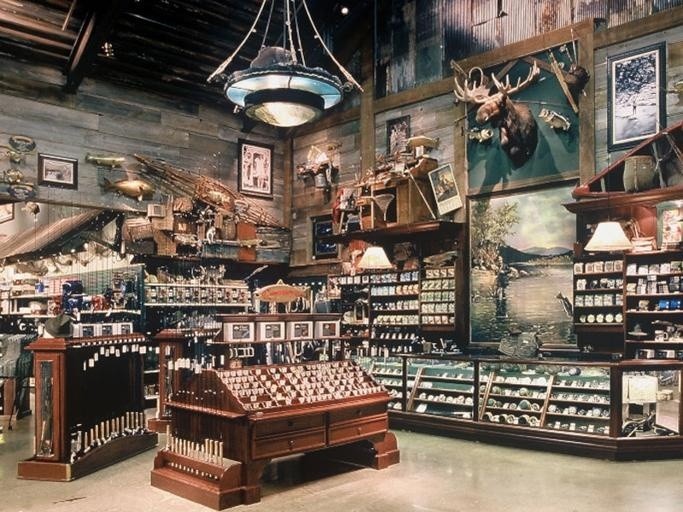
[44,312,74,339]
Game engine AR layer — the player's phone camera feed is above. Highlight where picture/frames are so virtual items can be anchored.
[237,138,273,200]
[606,40,665,153]
[37,152,78,191]
[0,202,15,224]
[465,177,580,351]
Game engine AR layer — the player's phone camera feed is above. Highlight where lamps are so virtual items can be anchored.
[207,0,364,128]
[582,153,634,252]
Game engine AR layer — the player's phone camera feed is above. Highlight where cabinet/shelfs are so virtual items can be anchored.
[353,156,439,231]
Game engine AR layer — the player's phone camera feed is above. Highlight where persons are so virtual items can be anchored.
[630,94,640,113]
[243,149,267,189]
[389,120,408,151]
[491,255,512,301]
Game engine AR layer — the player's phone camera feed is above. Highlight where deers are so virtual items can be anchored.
[453,60,542,168]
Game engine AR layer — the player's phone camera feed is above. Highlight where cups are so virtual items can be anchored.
[654,330,668,342]
